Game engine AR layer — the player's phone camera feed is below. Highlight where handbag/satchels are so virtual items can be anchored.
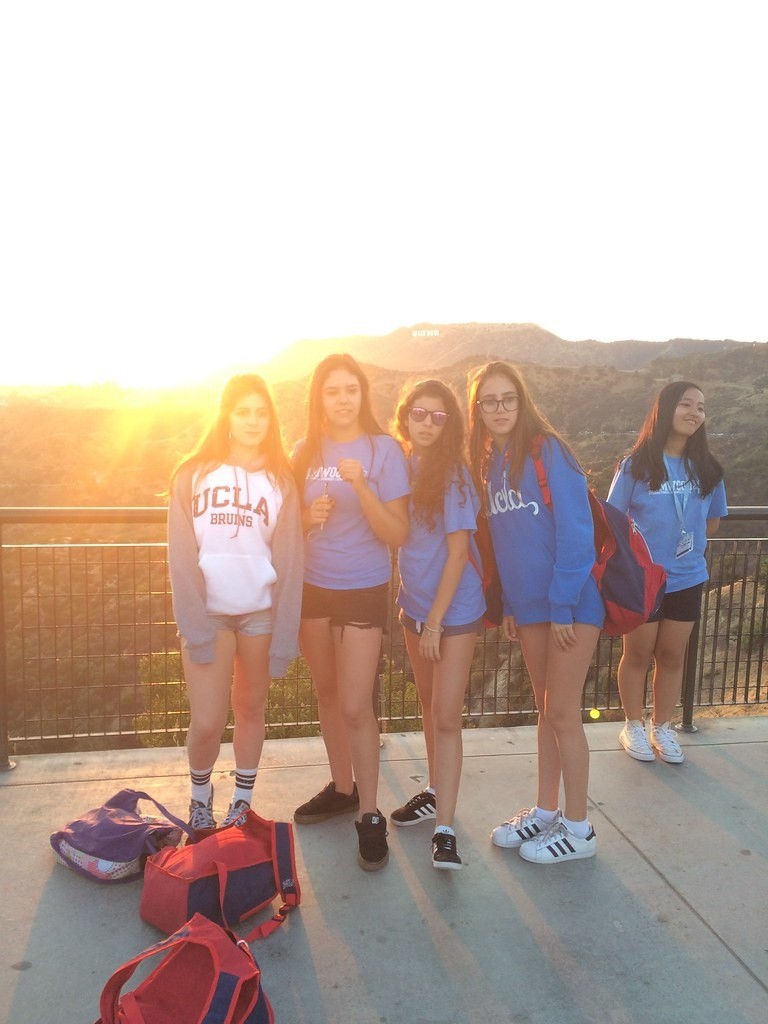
[49,788,197,883]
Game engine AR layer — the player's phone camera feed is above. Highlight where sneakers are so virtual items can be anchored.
[390,790,436,826]
[650,717,684,763]
[491,807,562,848]
[355,808,390,871]
[431,833,463,870]
[187,784,217,829]
[619,716,655,761]
[519,818,597,864]
[294,781,360,823]
[220,800,251,828]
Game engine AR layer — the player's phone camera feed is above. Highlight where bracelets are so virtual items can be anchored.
[422,625,445,634]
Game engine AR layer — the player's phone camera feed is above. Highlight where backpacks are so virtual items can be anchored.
[438,460,504,628]
[139,809,301,942]
[531,432,666,637]
[95,913,275,1024]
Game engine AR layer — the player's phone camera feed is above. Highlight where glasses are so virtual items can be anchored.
[476,396,520,414]
[407,406,450,427]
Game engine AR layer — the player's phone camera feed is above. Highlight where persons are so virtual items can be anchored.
[166,373,307,835]
[396,378,489,872]
[465,360,608,866]
[605,382,728,764]
[287,352,412,874]
